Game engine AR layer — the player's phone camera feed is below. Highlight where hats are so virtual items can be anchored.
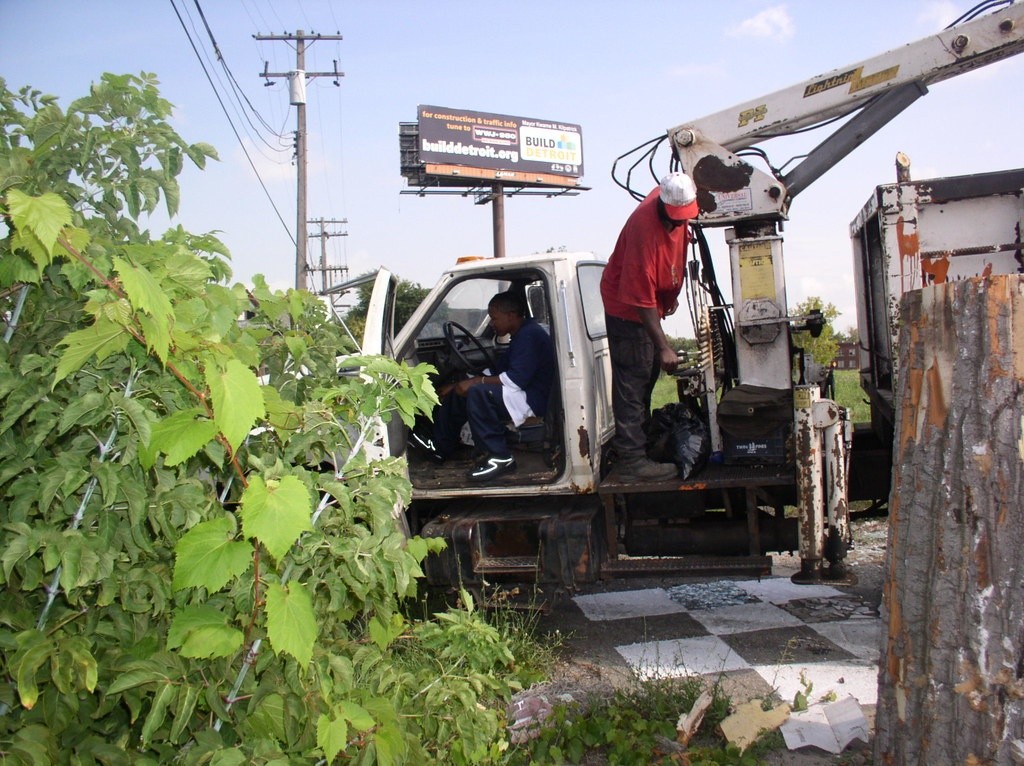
[660,172,698,220]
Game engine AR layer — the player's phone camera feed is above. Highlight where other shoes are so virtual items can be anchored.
[466,452,517,482]
[618,457,678,483]
[408,428,446,466]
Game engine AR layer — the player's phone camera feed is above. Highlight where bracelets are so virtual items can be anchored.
[481,376,484,384]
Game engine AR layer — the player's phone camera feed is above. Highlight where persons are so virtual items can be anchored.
[410,291,554,479]
[600,172,699,484]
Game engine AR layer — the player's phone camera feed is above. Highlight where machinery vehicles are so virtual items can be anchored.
[264,1,1024,613]
